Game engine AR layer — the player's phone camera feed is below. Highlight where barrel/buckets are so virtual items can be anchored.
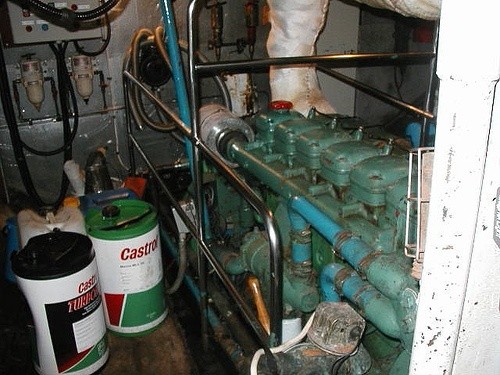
[12,199,169,375]
